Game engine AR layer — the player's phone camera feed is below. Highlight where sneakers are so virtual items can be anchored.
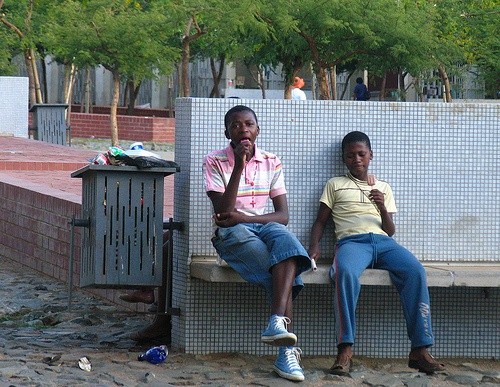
[272,346,305,381]
[260,314,297,347]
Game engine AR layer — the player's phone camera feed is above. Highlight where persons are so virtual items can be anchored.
[202,105,312,383]
[354,77,367,100]
[308,131,442,373]
[292,77,306,100]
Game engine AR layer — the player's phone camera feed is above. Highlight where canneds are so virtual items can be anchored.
[93,153,112,165]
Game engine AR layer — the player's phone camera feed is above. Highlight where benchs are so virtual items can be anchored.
[190,256,500,287]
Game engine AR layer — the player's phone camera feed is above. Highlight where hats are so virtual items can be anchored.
[289,77,305,89]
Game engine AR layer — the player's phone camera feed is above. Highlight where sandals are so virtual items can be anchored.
[409,349,447,376]
[329,350,355,375]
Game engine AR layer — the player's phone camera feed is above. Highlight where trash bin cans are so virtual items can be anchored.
[29,104,69,146]
[71,164,176,289]
